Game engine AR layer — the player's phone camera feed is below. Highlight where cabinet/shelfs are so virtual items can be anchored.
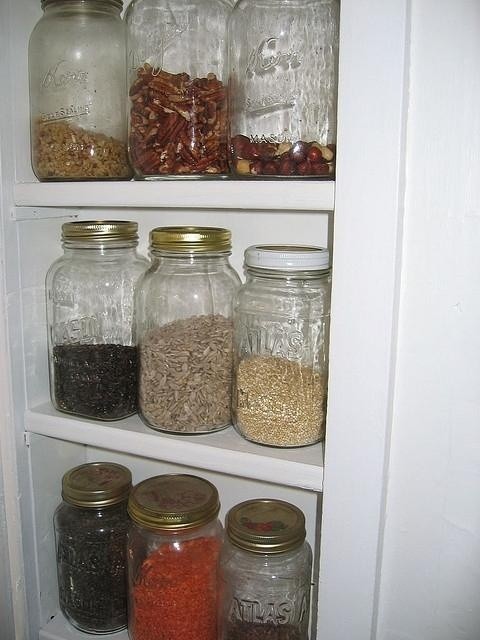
[0,0,411,640]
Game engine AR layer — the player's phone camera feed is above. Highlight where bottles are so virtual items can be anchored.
[27,1,340,182]
[44,218,333,449]
[52,459,314,640]
